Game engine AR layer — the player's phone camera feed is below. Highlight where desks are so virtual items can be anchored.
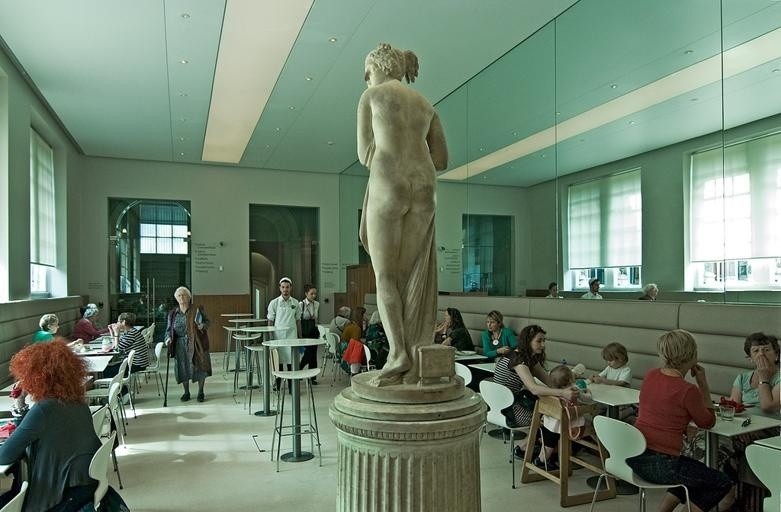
[688,412,780,470]
[754,436,780,450]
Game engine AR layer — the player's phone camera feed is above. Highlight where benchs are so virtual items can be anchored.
[557,291,780,305]
[363,292,642,495]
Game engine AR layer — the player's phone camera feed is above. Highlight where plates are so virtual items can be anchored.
[715,411,745,417]
[461,350,476,355]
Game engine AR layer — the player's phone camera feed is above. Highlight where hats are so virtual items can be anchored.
[588,277,599,285]
[280,277,292,285]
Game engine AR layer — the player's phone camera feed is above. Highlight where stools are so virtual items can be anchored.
[271,368,322,473]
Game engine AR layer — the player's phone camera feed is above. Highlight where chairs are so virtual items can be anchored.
[316,323,376,387]
[745,444,781,511]
[519,396,617,508]
[0,322,167,512]
[589,414,692,511]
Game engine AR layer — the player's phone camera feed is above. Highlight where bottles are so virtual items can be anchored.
[558,358,568,367]
[100,336,110,353]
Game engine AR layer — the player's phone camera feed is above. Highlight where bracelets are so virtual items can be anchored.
[115,335,119,337]
[9,402,29,413]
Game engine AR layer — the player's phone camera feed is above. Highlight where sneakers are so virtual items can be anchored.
[122,390,135,405]
[308,379,317,385]
[532,456,560,471]
[180,392,190,401]
[273,382,280,391]
[513,445,526,461]
[196,393,204,402]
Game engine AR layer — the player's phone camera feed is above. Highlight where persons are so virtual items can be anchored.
[72,302,112,344]
[104,312,148,405]
[298,285,321,385]
[267,276,302,394]
[637,283,659,300]
[164,285,212,402]
[0,334,106,511]
[357,41,448,384]
[580,277,601,299]
[32,313,60,345]
[546,282,564,298]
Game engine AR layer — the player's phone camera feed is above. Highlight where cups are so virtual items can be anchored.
[718,404,734,422]
[494,357,502,369]
[72,344,82,354]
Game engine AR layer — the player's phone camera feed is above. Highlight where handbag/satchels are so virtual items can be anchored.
[167,338,175,358]
[517,389,539,411]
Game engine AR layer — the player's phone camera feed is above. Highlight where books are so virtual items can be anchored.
[195,308,203,334]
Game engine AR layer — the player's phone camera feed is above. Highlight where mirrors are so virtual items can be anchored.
[556,0,725,305]
[467,18,558,298]
[433,81,467,297]
[721,0,780,306]
[339,160,371,264]
